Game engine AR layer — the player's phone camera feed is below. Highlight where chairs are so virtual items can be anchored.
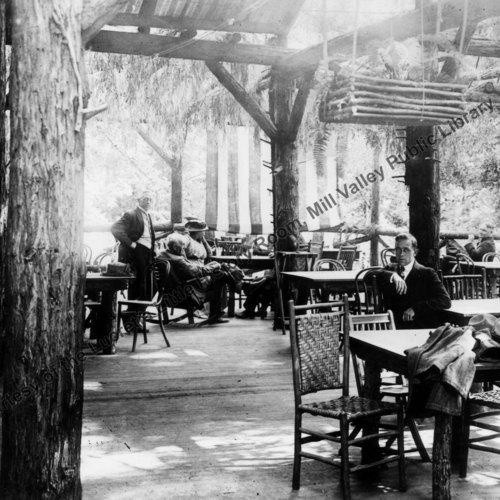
[84,232,499,500]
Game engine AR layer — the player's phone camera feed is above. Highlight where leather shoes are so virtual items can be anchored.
[207,316,229,325]
[253,309,267,317]
[132,317,150,334]
[241,276,267,297]
[121,310,131,335]
[235,309,256,320]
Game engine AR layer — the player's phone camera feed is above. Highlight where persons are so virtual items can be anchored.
[160,239,237,323]
[232,241,278,320]
[182,216,214,266]
[362,232,452,331]
[439,220,497,300]
[164,222,185,249]
[111,190,156,335]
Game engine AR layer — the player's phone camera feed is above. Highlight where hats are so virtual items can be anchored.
[467,315,497,337]
[184,219,209,232]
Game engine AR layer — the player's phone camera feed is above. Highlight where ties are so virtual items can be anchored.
[396,264,405,279]
